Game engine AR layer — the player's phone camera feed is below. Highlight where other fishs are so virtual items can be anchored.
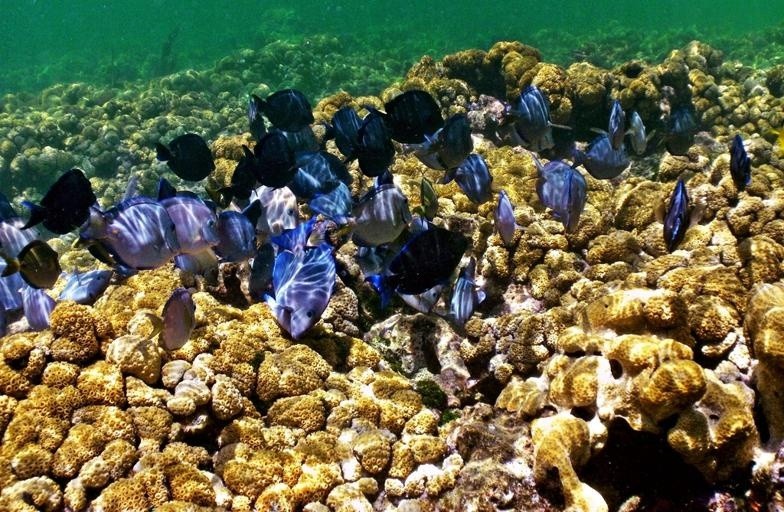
[0,90,493,354]
